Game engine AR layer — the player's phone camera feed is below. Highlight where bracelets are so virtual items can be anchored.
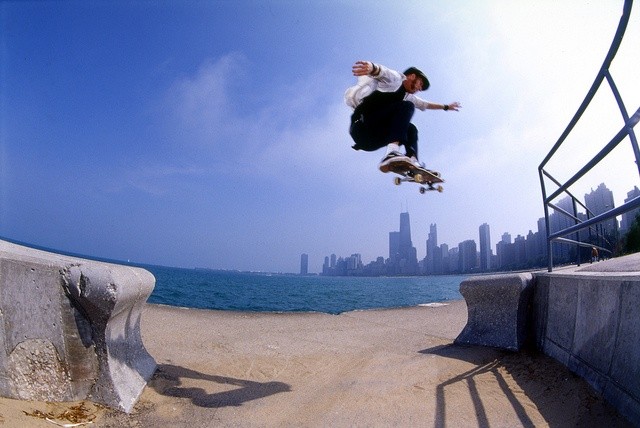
[444,104,449,111]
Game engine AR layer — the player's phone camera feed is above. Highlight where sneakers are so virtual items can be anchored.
[404,152,426,168]
[379,151,413,173]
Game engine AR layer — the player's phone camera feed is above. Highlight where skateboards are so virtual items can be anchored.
[379,160,445,194]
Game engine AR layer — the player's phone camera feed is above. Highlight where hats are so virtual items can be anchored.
[402,66,430,90]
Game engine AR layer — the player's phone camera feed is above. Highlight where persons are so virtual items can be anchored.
[342,59,463,177]
